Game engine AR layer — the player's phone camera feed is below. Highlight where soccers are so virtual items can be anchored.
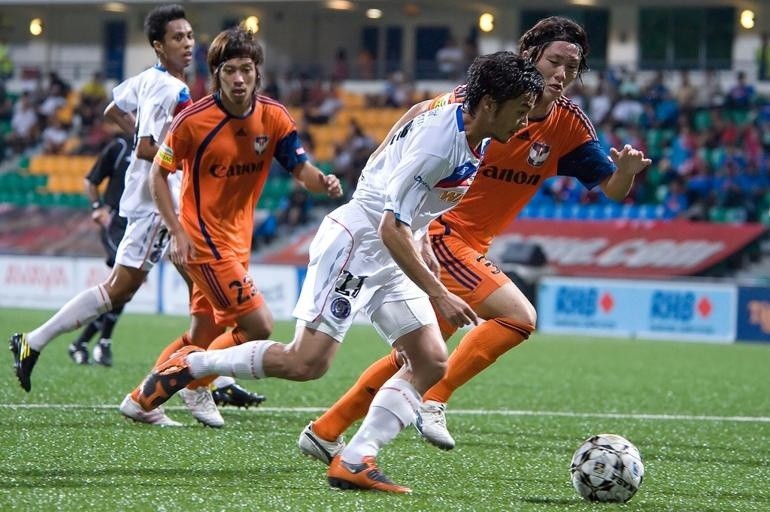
[570,434,644,502]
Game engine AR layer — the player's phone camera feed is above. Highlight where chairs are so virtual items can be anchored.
[2,81,769,235]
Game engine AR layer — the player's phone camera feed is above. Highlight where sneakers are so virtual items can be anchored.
[412,401,456,450]
[93,338,111,366]
[299,423,412,493]
[8,333,40,392]
[119,345,265,427]
[70,342,86,364]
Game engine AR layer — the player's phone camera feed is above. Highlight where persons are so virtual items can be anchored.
[297,16,652,468]
[120,27,343,429]
[9,4,267,407]
[68,110,140,368]
[1,33,769,279]
[135,52,547,495]
